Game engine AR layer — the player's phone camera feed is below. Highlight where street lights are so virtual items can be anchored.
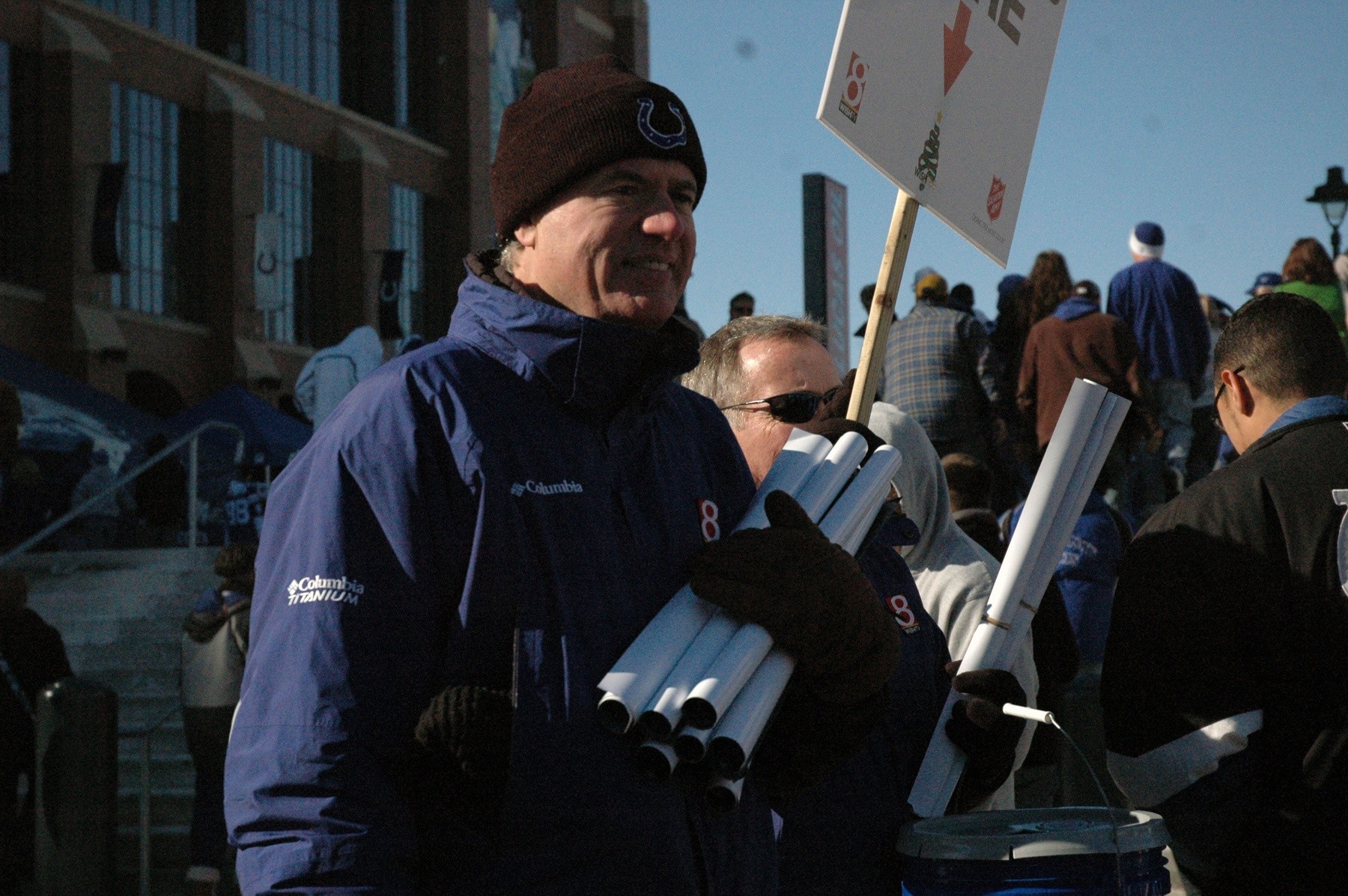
[1303,164,1348,270]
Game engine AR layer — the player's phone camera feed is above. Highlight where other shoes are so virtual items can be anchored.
[1163,466,1186,500]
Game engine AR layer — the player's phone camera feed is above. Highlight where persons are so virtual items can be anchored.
[1,54,1348,896]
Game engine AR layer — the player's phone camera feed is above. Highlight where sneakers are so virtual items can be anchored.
[187,866,224,883]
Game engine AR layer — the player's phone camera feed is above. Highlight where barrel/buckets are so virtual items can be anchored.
[898,702,1173,896]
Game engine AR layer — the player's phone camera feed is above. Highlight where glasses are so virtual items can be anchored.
[720,389,839,424]
[735,306,754,314]
[1207,364,1248,433]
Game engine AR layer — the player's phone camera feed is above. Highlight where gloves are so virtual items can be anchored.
[405,687,518,834]
[694,491,877,668]
[944,670,1027,815]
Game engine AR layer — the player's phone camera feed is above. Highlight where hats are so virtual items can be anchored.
[1248,273,1285,294]
[1072,280,1101,299]
[915,268,947,298]
[1129,222,1167,260]
[491,52,707,240]
